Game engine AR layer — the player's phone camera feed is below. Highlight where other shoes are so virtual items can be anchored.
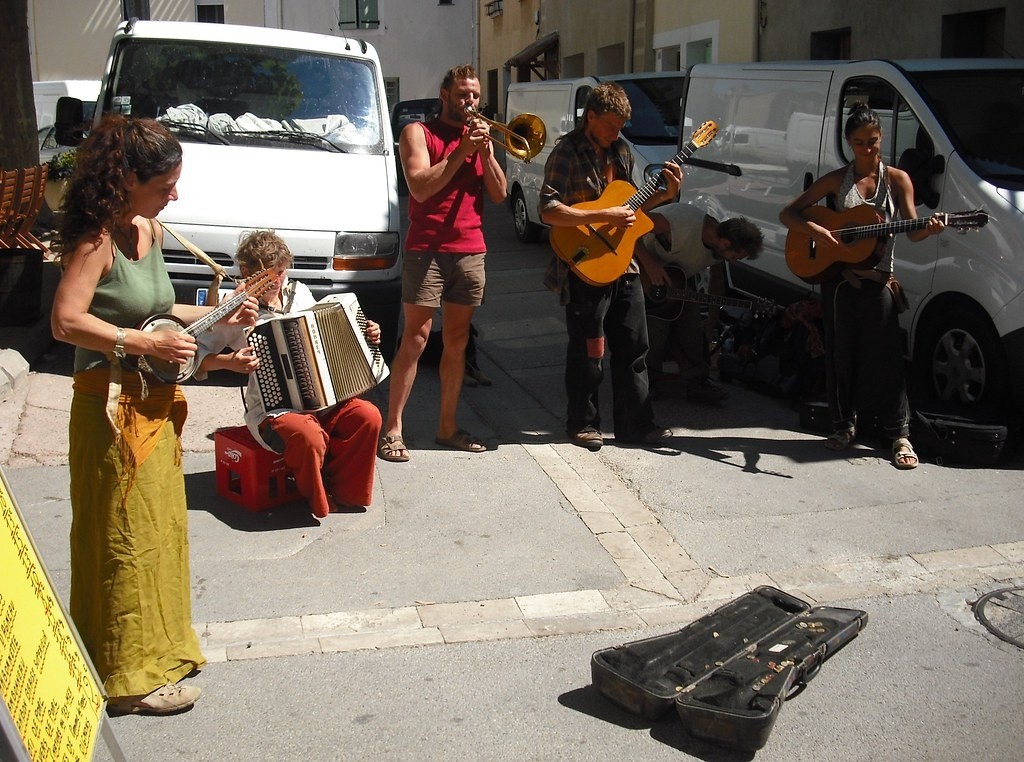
[614,426,673,441]
[573,432,603,447]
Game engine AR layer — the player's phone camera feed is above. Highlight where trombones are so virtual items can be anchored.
[464,105,547,164]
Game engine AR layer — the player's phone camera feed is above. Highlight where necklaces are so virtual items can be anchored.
[115,223,135,252]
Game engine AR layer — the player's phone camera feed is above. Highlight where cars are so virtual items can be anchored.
[37,125,88,166]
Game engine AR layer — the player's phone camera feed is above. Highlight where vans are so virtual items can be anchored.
[34,80,101,128]
[505,69,688,243]
[53,17,401,357]
[677,58,1024,420]
[389,98,442,187]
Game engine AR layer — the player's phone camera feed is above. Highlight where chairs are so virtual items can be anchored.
[964,102,1024,184]
[20,162,48,253]
[897,93,959,142]
[0,169,19,248]
[195,64,249,120]
[280,70,341,123]
[8,165,48,260]
[131,68,180,118]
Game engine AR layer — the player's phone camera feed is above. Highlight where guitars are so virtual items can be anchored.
[133,268,280,384]
[785,204,988,285]
[640,265,777,322]
[548,119,720,286]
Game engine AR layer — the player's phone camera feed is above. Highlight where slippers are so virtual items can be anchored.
[892,438,919,468]
[825,426,857,450]
[435,430,487,451]
[379,436,410,461]
[108,683,201,714]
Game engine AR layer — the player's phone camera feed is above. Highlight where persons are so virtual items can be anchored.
[379,63,508,461]
[535,81,685,447]
[50,113,260,713]
[191,229,383,518]
[635,201,763,403]
[779,102,946,470]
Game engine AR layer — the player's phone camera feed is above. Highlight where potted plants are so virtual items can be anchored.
[42,148,77,211]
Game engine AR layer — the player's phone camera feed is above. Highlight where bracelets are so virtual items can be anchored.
[113,327,127,359]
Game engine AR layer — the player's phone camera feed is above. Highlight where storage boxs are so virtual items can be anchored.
[214,426,305,513]
